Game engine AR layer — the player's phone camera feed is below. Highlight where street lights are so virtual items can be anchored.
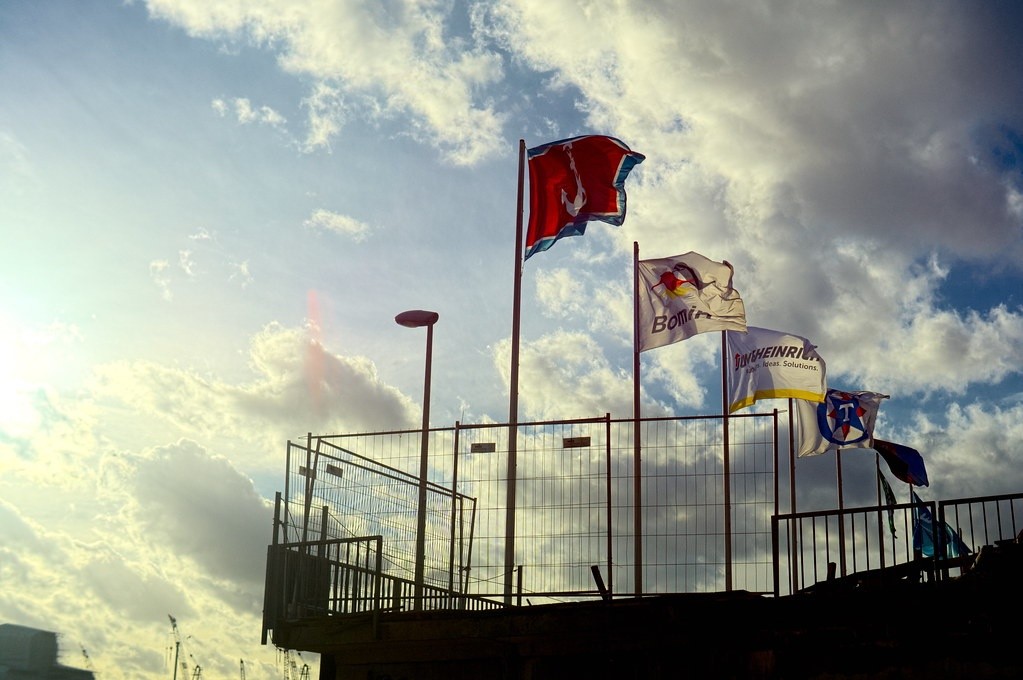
[395,308,439,612]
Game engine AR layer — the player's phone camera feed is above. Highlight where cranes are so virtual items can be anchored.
[167,612,202,680]
[239,659,246,680]
[283,647,312,680]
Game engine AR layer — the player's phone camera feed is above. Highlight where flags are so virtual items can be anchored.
[726,324,826,412]
[796,388,888,458]
[873,439,928,487]
[523,134,645,260]
[637,251,747,352]
[912,493,971,561]
[879,470,897,538]
